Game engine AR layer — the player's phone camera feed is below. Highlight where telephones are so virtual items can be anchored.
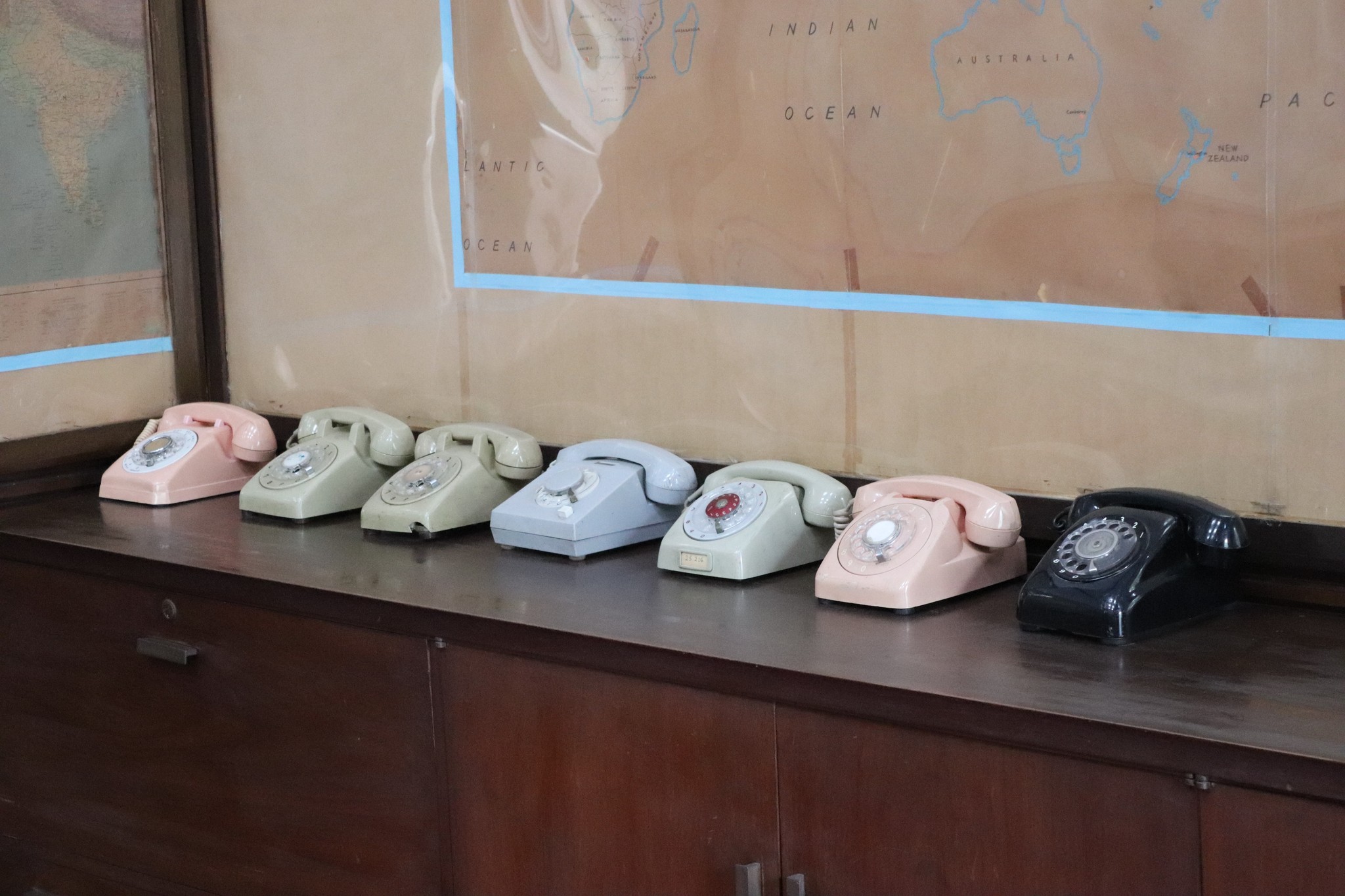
[98,402,278,508]
[813,473,1029,620]
[490,436,697,565]
[655,458,854,587]
[238,406,416,524]
[1013,486,1245,647]
[359,422,547,542]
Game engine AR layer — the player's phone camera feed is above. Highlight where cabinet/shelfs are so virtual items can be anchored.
[1,481,1345,896]
